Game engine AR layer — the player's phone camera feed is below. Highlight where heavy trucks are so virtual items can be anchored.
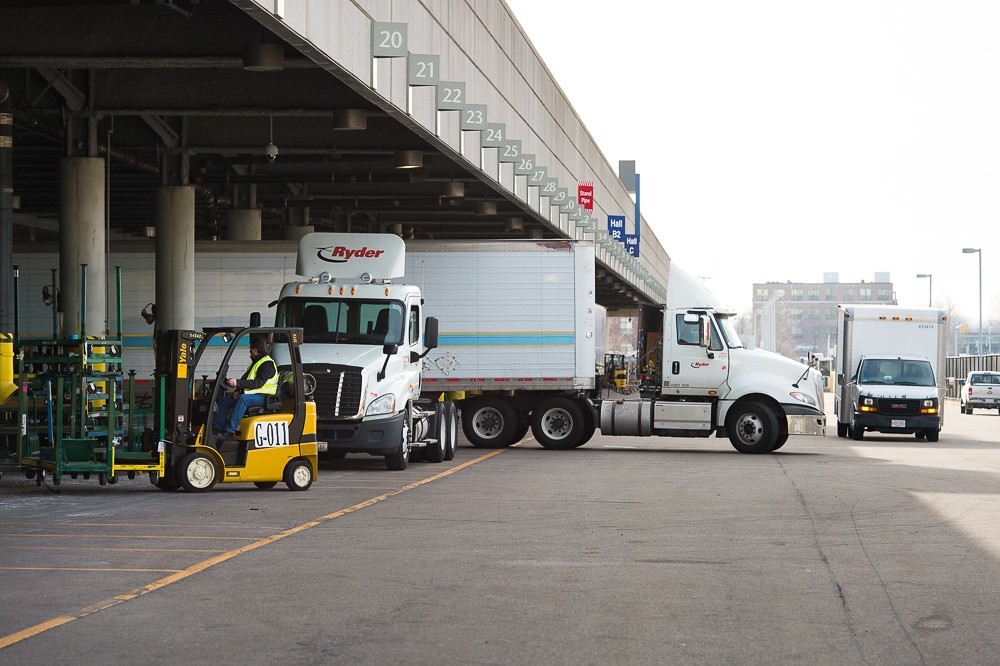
[9,237,823,455]
[248,232,459,471]
[833,303,955,443]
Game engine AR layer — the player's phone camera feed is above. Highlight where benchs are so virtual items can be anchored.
[974,378,999,384]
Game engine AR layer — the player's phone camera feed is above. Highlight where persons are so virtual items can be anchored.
[211,337,279,440]
[851,362,884,382]
[905,365,923,378]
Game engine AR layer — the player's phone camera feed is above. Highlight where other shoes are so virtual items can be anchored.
[212,426,224,434]
[219,431,234,440]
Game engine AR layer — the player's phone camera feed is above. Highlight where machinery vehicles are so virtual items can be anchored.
[88,323,319,495]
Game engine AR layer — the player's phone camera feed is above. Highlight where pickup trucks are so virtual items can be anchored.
[957,371,1000,414]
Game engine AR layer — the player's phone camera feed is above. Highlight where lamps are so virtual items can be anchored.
[596,268,640,302]
[503,206,523,230]
[476,185,497,215]
[242,27,284,71]
[444,164,465,197]
[396,132,423,169]
[331,86,368,131]
[527,221,543,239]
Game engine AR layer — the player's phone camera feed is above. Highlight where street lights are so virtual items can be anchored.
[962,247,983,371]
[917,273,932,308]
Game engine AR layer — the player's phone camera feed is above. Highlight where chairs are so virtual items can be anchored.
[375,309,402,336]
[244,379,288,418]
[300,306,329,336]
[901,363,923,376]
